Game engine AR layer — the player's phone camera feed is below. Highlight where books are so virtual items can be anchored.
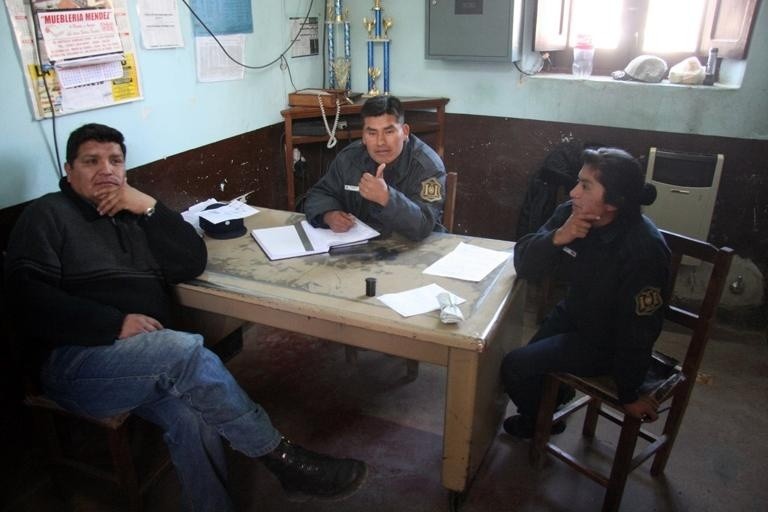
[330,240,369,249]
[249,212,379,263]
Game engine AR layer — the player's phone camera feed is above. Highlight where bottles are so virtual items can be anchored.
[572,43,594,78]
[706,47,719,75]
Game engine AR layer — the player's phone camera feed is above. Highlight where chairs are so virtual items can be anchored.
[527,227,734,510]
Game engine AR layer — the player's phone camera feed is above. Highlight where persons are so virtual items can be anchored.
[494,146,676,440]
[0,120,367,512]
[301,92,449,244]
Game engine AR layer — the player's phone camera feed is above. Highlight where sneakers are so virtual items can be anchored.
[503,382,579,439]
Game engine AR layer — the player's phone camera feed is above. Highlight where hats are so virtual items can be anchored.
[198,203,248,240]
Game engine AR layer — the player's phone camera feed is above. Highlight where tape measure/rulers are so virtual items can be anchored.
[295,221,314,251]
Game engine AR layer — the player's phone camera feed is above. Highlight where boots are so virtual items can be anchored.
[263,431,369,504]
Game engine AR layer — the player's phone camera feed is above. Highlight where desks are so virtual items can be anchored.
[163,196,527,495]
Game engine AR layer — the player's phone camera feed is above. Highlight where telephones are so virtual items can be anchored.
[296,90,331,95]
